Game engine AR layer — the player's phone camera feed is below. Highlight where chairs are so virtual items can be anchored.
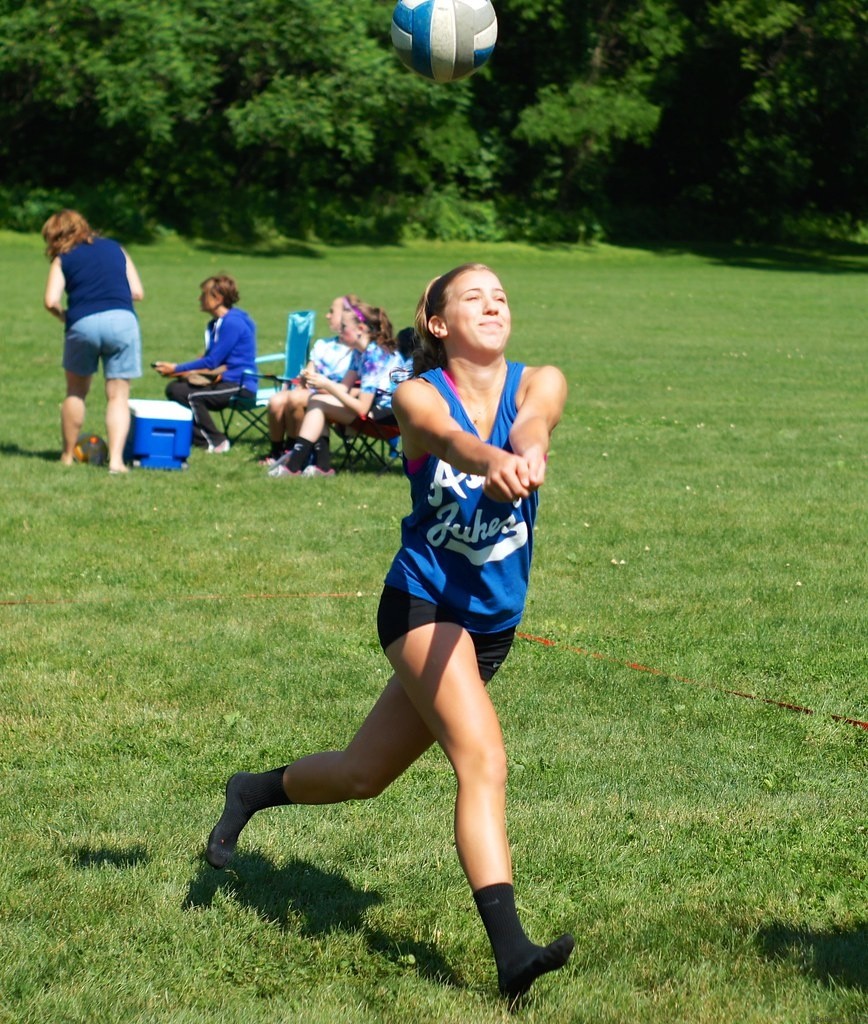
[220,309,315,446]
[333,414,406,472]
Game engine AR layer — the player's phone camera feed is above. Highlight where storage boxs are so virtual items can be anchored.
[122,399,193,471]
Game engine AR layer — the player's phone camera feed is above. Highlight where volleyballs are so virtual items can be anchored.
[391,1,500,84]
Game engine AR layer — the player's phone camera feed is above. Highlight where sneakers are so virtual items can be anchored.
[259,457,280,465]
[303,465,335,477]
[268,464,302,477]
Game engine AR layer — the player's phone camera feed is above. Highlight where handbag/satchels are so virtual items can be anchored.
[186,373,222,386]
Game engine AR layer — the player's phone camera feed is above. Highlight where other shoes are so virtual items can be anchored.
[206,437,230,452]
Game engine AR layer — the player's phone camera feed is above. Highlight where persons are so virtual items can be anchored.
[204,262,575,997]
[152,274,260,454]
[41,208,144,474]
[258,294,421,479]
